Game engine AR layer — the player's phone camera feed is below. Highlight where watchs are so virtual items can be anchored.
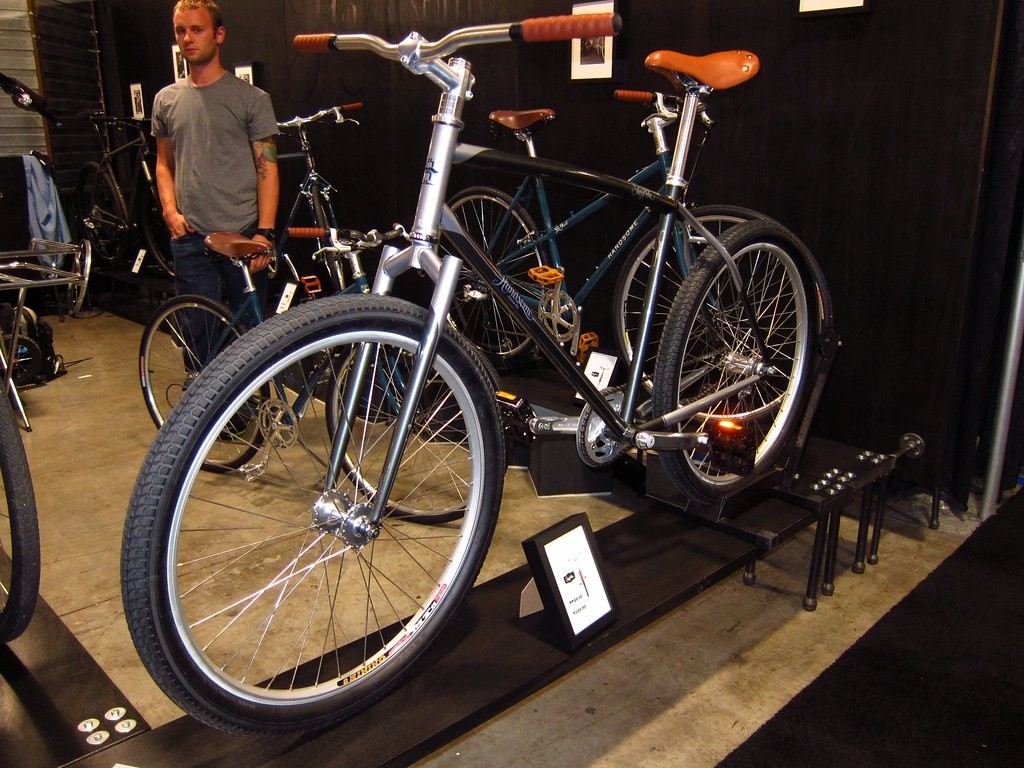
[255,227,277,242]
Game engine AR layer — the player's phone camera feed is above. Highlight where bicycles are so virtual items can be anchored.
[265,103,367,293]
[434,80,809,422]
[133,227,507,524]
[0,74,66,639]
[76,112,181,277]
[120,11,840,738]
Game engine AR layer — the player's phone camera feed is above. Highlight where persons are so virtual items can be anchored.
[177,53,185,78]
[149,1,283,441]
[585,36,596,54]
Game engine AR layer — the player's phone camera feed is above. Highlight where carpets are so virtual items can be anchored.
[714,485,1024,768]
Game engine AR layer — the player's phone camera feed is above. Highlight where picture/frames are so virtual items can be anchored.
[790,0,870,19]
[569,0,621,84]
[232,61,256,88]
[169,41,190,84]
[518,512,623,651]
[130,83,145,120]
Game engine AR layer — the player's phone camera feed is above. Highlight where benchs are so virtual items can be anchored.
[1,544,152,768]
[81,261,895,614]
[62,502,767,766]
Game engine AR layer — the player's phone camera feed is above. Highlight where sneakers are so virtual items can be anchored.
[218,414,255,439]
